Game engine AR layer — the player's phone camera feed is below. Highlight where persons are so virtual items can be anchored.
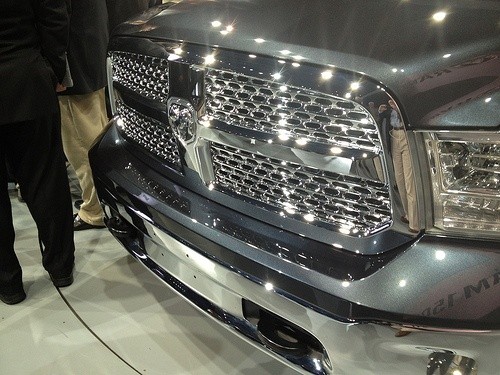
[54,0,112,232]
[0,0,75,305]
[105,1,162,122]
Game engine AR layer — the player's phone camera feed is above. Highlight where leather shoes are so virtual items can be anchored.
[73,199,84,209]
[73,214,108,232]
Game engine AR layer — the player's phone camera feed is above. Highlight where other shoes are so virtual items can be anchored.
[0,286,27,306]
[49,262,74,286]
